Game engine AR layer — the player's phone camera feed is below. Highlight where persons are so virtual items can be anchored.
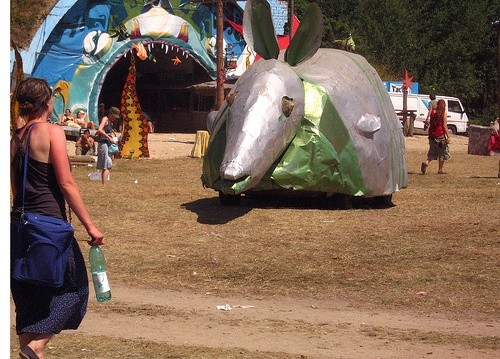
[426,94,438,120]
[421,99,451,175]
[10,78,105,359]
[60,104,153,184]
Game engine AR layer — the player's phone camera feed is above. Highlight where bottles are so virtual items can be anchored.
[71,117,73,121]
[89,245,111,301]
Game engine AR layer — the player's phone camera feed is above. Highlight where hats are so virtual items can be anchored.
[109,106,120,119]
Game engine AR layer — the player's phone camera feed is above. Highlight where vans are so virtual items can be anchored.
[388,92,468,135]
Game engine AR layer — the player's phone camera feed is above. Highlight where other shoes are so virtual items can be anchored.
[18,344,40,359]
[438,170,448,174]
[421,162,428,174]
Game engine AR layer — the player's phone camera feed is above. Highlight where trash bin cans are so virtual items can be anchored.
[467,125,494,155]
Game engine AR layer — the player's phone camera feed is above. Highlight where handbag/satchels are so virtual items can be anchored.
[10,212,74,293]
